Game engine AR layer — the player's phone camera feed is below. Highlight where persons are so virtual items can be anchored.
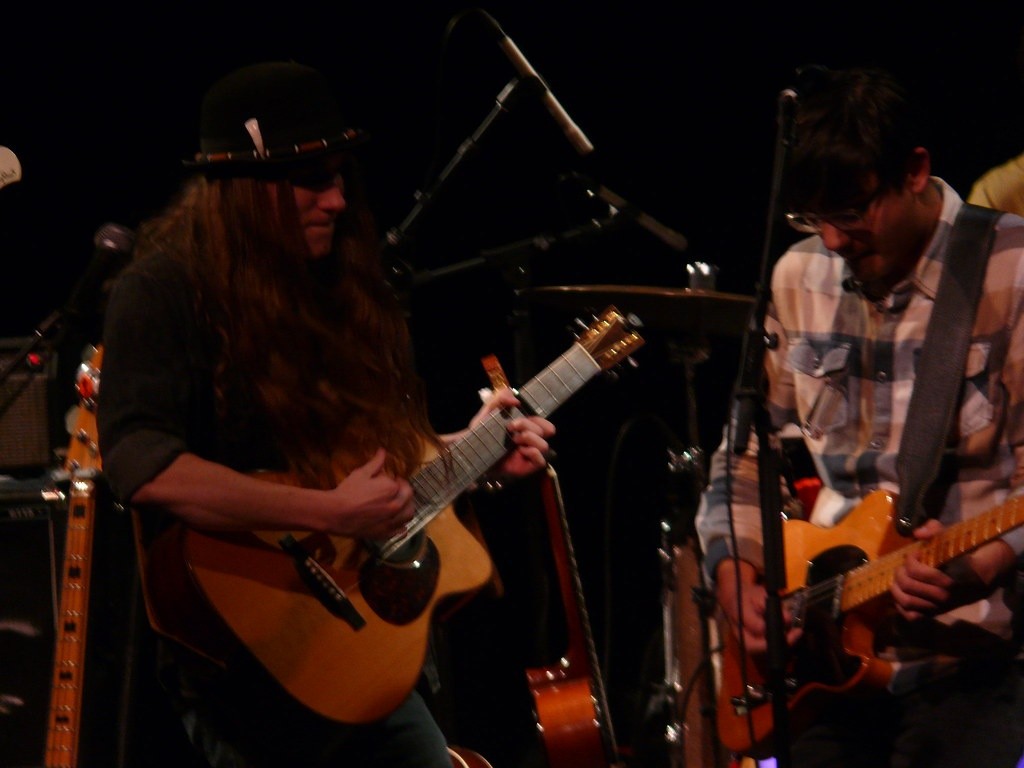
[97,60,558,768]
[695,57,1023,768]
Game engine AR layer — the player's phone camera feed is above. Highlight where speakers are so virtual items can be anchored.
[0,335,69,472]
[0,499,96,768]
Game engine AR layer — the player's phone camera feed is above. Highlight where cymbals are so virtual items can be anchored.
[530,275,759,334]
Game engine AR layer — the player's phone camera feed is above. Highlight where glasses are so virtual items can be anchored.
[783,179,894,234]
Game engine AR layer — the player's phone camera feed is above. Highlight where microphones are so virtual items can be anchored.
[476,10,594,153]
[64,222,134,317]
[575,171,687,250]
[777,88,797,146]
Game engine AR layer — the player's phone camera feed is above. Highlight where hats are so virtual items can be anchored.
[183,62,369,177]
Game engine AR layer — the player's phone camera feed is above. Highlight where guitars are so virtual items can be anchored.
[135,304,645,731]
[37,343,106,768]
[474,346,628,766]
[709,485,1024,750]
